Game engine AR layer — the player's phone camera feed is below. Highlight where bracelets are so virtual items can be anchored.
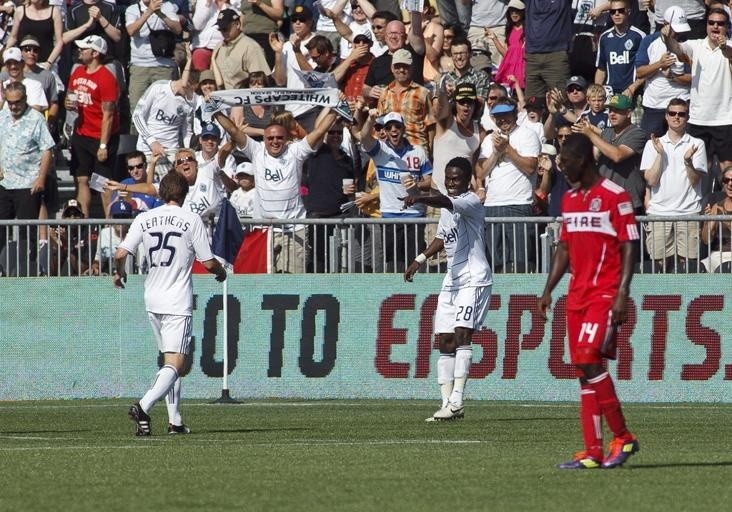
[113,270,124,275]
[413,252,426,266]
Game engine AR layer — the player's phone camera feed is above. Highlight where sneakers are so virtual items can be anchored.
[424,402,465,423]
[167,423,194,435]
[128,402,152,436]
[603,436,640,469]
[557,453,602,469]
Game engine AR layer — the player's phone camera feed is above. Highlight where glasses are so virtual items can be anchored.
[608,7,627,14]
[666,109,687,118]
[568,86,583,93]
[372,24,385,30]
[375,125,403,132]
[706,18,728,26]
[351,4,359,8]
[443,34,454,40]
[267,135,284,141]
[127,162,143,171]
[291,17,309,24]
[489,95,507,100]
[176,156,196,166]
[327,128,344,134]
[556,135,568,141]
[721,176,731,184]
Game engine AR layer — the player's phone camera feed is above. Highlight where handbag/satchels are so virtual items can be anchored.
[149,29,176,58]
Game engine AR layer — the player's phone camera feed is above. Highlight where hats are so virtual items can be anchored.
[111,201,132,217]
[491,102,516,115]
[373,111,404,126]
[211,9,240,27]
[455,82,477,102]
[199,69,215,85]
[523,96,544,109]
[202,122,220,138]
[290,4,312,18]
[603,94,633,110]
[663,6,692,34]
[392,49,415,67]
[236,161,254,176]
[74,35,108,56]
[351,26,371,41]
[2,35,41,65]
[505,0,526,11]
[564,75,588,90]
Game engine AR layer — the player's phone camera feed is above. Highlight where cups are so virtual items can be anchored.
[123,191,132,202]
[399,171,411,188]
[379,84,386,91]
[67,93,79,108]
[342,177,353,195]
[354,193,365,208]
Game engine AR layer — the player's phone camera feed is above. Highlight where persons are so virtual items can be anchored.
[537,133,643,469]
[111,167,228,435]
[395,156,494,422]
[0,1,732,276]
